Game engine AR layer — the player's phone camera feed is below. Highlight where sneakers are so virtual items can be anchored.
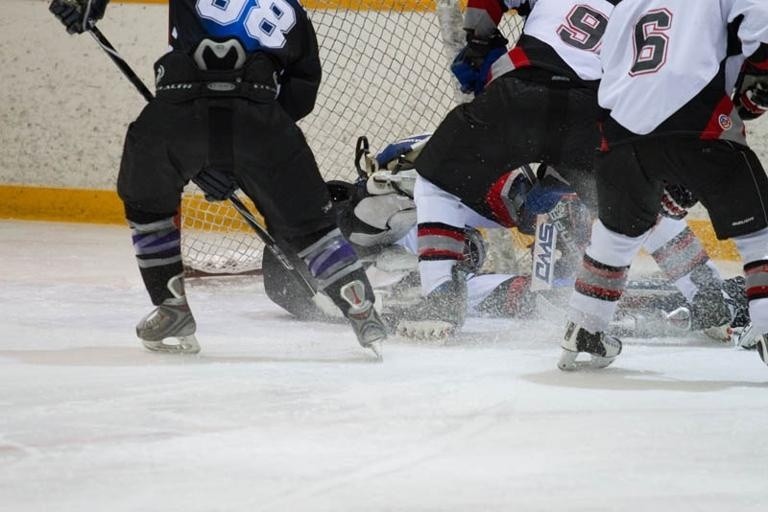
[135,270,196,341]
[754,331,768,367]
[340,280,389,346]
[560,320,624,358]
[401,265,468,328]
[691,264,732,330]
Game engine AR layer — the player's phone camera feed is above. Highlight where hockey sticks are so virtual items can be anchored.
[88,25,384,317]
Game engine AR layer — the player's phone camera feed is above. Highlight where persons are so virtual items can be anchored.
[320,129,693,326]
[560,0,768,365]
[49,0,386,347]
[378,0,732,332]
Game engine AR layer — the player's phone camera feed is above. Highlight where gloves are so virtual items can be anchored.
[47,0,108,35]
[524,163,577,216]
[658,184,699,221]
[735,82,767,121]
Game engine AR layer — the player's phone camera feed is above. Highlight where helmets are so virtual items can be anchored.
[465,227,489,270]
